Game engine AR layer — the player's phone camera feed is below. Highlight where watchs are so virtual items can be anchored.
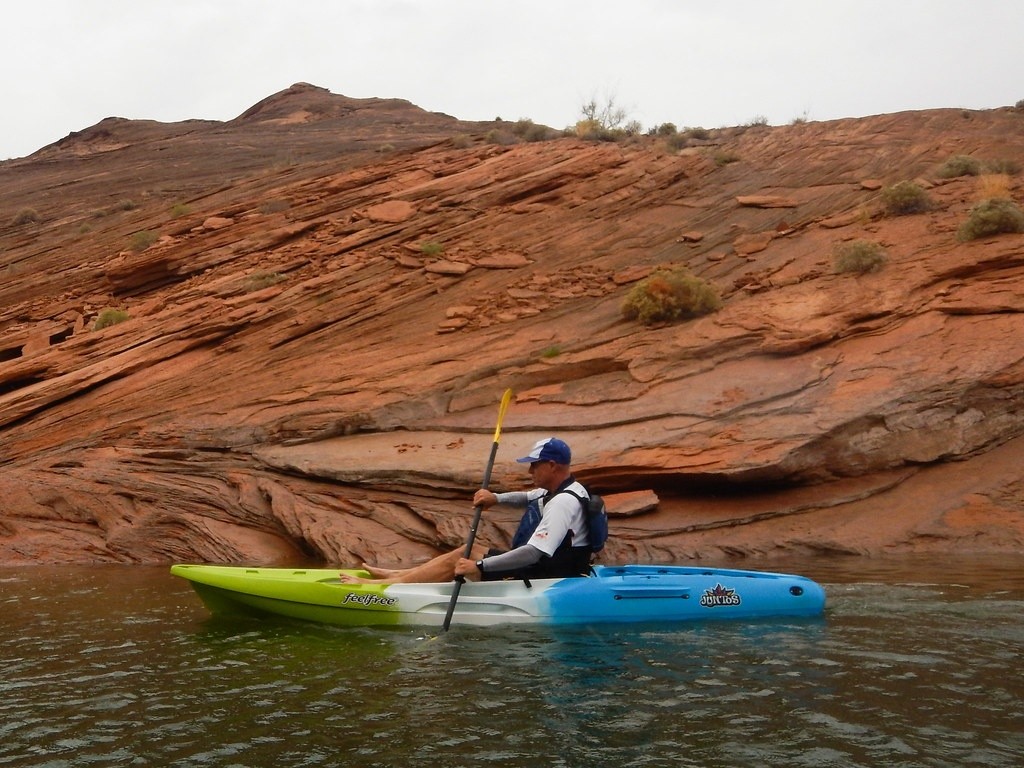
[476,560,484,572]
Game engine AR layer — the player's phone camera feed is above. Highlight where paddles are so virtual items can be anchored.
[442,389,513,631]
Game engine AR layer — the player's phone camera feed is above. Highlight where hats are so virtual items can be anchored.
[516,437,572,465]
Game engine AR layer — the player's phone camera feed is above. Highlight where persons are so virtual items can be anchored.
[339,438,591,584]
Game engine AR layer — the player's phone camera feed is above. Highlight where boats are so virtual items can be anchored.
[169,562,828,628]
